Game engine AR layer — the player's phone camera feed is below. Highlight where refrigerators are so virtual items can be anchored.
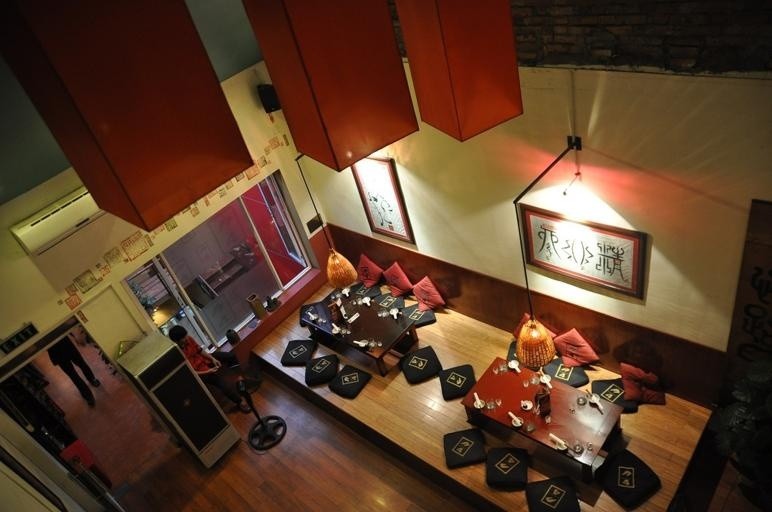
[116,330,241,470]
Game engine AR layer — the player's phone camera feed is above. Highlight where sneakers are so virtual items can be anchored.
[235,379,246,392]
[236,402,250,412]
[84,377,101,408]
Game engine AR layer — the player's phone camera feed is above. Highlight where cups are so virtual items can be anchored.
[573,439,583,452]
[486,398,495,410]
[576,390,586,405]
[498,358,508,372]
[493,366,499,375]
[523,380,529,388]
[495,399,502,406]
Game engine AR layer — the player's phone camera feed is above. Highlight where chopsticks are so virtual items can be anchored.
[538,369,552,389]
[549,433,579,455]
[586,389,604,414]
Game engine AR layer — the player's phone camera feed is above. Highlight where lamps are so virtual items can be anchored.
[294,154,357,289]
[513,133,583,366]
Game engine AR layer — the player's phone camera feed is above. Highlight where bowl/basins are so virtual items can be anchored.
[358,339,369,347]
[309,313,318,320]
[342,288,350,295]
[390,307,399,316]
[588,393,600,404]
[332,327,341,334]
[540,374,551,383]
[508,360,519,369]
[362,296,371,304]
[474,399,485,409]
[512,418,524,427]
[557,439,568,450]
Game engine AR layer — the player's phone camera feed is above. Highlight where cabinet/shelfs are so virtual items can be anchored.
[200,251,245,297]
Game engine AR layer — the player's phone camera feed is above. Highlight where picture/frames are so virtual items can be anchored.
[519,201,651,301]
[350,157,414,243]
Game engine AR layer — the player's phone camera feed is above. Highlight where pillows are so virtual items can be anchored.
[552,328,599,367]
[620,362,667,405]
[357,253,382,289]
[383,261,413,298]
[513,313,558,341]
[412,276,446,312]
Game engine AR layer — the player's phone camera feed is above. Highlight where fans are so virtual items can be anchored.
[222,363,287,451]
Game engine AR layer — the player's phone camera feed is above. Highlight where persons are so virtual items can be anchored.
[47,335,101,407]
[168,324,264,414]
